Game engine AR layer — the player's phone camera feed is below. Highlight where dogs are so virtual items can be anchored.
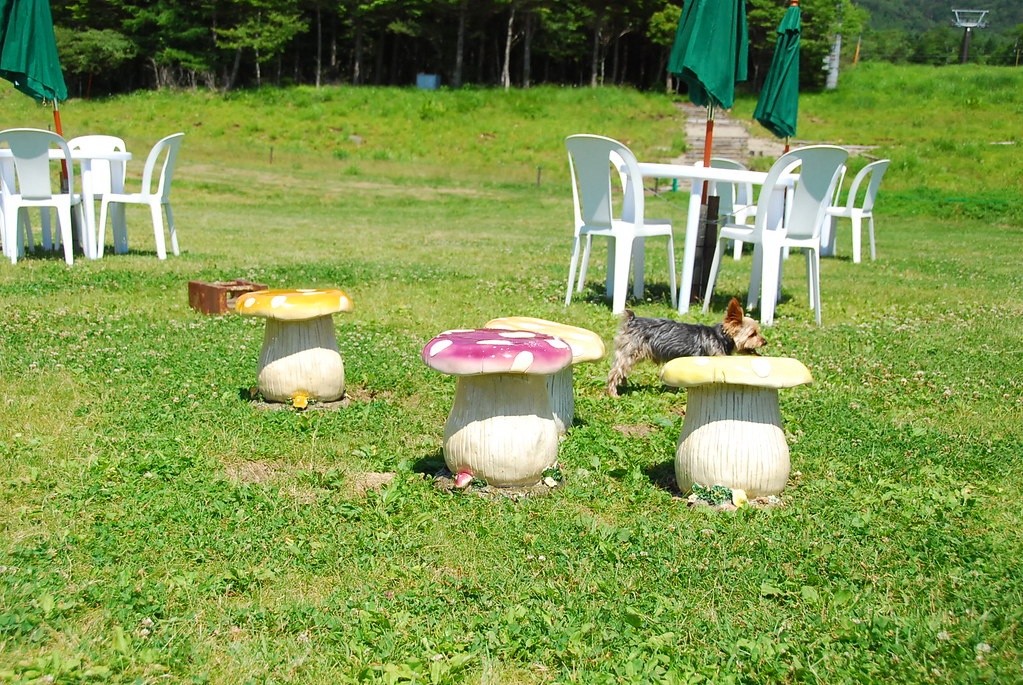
[605,296,767,403]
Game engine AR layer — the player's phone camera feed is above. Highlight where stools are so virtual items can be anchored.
[231,286,354,404]
[484,316,605,437]
[422,327,573,489]
[660,354,814,503]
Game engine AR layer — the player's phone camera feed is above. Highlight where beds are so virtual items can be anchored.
[563,133,678,312]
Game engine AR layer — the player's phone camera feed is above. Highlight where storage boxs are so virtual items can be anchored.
[415,72,441,90]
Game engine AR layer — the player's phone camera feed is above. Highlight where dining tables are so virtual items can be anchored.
[0,148,132,254]
[618,162,795,312]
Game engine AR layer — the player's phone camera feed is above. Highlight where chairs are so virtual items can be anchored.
[96,132,186,260]
[700,143,850,330]
[820,165,848,256]
[0,127,90,265]
[53,133,127,253]
[694,158,760,261]
[819,158,892,266]
